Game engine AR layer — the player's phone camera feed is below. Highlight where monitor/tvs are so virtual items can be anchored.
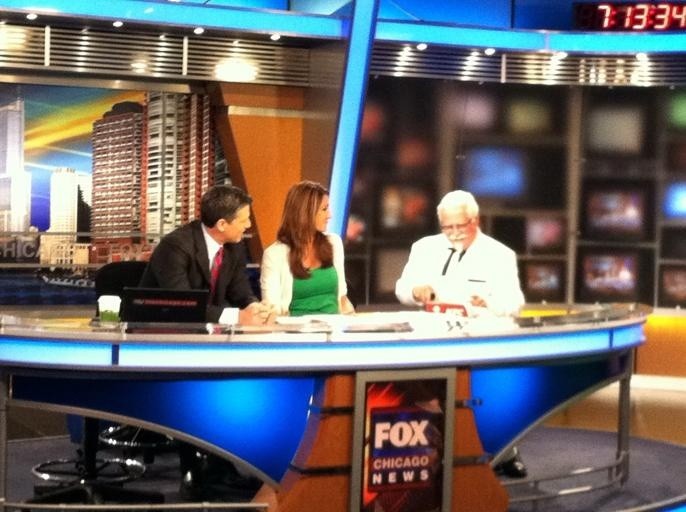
[120,286,211,324]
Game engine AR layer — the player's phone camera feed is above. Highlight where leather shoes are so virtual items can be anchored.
[504,458,530,480]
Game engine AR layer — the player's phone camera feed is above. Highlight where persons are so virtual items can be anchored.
[260,179,355,317]
[394,190,527,318]
[139,185,283,327]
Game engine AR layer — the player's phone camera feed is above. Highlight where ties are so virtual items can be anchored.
[209,248,225,304]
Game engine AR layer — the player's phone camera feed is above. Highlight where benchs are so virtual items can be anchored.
[536,303,686,445]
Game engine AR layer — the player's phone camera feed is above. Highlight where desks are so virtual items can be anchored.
[1,300,655,510]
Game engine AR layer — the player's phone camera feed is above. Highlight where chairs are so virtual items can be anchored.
[221,265,263,304]
[97,259,186,450]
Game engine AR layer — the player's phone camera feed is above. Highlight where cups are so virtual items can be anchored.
[96,295,122,320]
